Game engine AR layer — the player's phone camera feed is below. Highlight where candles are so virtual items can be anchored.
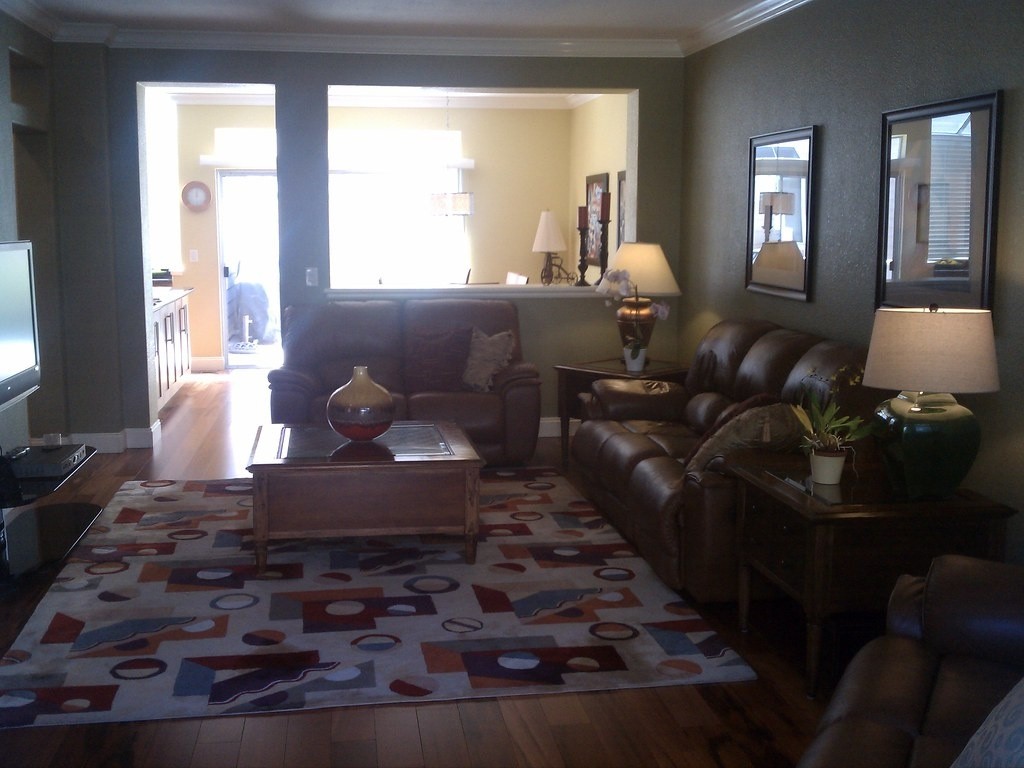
[577,205,588,228]
[599,192,610,221]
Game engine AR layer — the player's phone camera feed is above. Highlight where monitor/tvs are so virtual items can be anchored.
[0,239,42,412]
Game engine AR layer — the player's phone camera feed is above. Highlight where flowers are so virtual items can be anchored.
[606,270,644,358]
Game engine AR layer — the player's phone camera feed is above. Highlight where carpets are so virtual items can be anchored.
[0,469,757,744]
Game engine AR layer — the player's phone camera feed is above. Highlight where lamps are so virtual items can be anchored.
[532,210,569,288]
[861,300,999,502]
[753,240,805,293]
[595,241,682,349]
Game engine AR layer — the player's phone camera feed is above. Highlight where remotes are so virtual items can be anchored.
[8,446,29,460]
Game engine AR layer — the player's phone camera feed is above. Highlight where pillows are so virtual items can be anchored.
[330,326,521,390]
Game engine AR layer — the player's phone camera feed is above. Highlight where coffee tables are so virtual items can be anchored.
[248,423,486,571]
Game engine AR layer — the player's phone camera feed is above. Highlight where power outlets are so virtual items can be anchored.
[190,249,198,262]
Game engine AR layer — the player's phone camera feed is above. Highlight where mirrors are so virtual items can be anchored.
[745,126,812,301]
[879,90,998,316]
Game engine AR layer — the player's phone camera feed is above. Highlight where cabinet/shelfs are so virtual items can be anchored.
[152,295,191,410]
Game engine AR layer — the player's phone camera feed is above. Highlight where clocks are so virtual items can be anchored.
[181,181,212,211]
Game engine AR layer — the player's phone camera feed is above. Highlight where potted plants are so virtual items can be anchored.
[793,385,870,484]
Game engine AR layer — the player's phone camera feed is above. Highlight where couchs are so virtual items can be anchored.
[793,555,1024,768]
[571,318,900,619]
[269,299,538,472]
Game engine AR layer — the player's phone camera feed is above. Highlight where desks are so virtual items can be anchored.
[732,456,1018,701]
[554,358,690,464]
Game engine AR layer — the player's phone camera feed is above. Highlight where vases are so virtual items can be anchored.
[623,347,647,372]
[327,366,395,444]
